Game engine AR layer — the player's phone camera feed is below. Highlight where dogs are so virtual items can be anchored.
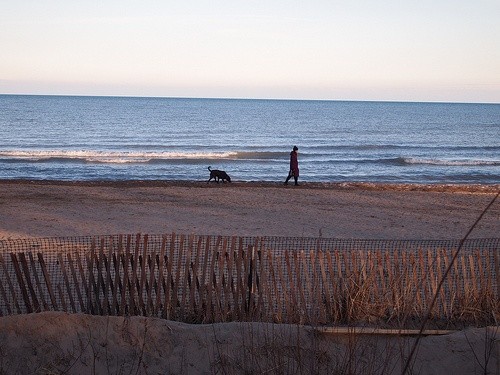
[207,166,231,184]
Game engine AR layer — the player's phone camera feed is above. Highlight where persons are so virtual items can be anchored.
[283,146,301,187]
